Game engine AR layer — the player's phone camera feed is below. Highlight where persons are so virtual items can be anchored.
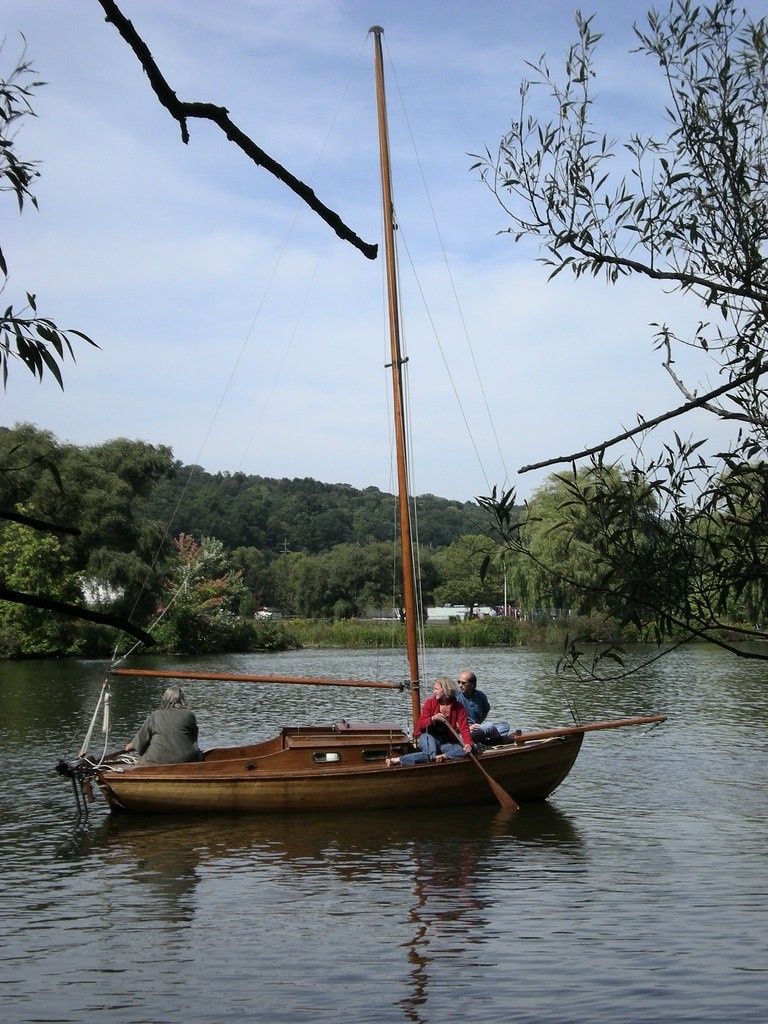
[123,684,199,766]
[497,607,505,616]
[385,673,477,767]
[454,671,510,742]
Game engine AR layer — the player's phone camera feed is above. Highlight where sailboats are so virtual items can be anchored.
[53,22,674,821]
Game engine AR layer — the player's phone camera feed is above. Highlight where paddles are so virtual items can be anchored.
[444,718,519,810]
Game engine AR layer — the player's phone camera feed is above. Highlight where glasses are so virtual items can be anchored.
[457,681,466,685]
